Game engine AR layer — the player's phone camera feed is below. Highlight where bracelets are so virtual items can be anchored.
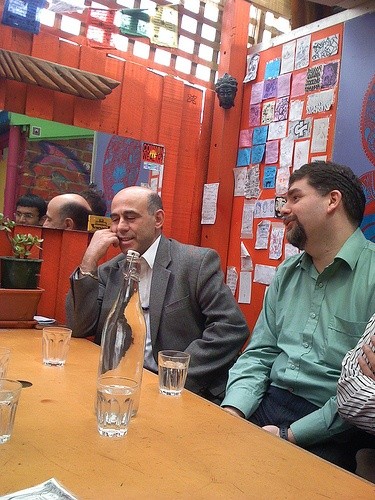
[279,423,290,440]
[79,267,95,277]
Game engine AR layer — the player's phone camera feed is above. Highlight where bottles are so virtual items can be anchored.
[96,250,147,418]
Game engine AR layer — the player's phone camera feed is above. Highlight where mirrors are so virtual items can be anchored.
[0,110,166,234]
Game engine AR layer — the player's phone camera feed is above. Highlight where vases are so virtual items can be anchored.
[0,287,46,328]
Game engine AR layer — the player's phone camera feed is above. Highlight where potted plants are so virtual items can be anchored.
[0,214,44,290]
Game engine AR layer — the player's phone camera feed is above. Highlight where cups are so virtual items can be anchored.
[0,379,22,444]
[42,327,72,367]
[0,348,11,388]
[159,351,190,397]
[96,378,138,438]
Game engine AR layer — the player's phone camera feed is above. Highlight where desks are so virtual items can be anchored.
[0,324,375,500]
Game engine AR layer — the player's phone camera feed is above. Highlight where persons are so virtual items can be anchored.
[215,73,238,109]
[65,187,250,397]
[14,190,107,231]
[337,313,375,437]
[220,159,375,474]
